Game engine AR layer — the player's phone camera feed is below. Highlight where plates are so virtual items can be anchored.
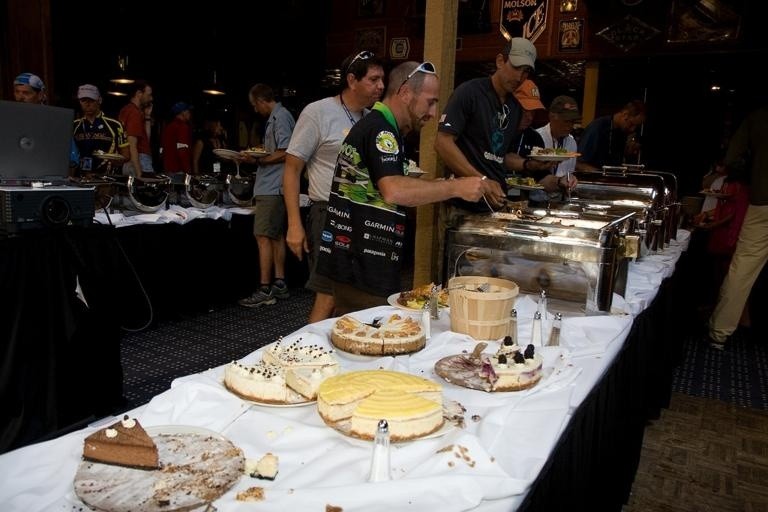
[410,396,464,439]
[407,172,430,177]
[94,153,124,161]
[432,352,543,393]
[240,397,317,408]
[75,423,246,511]
[328,336,427,358]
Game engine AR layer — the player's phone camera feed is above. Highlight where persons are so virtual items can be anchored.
[13,72,80,176]
[698,107,768,352]
[74,80,228,181]
[232,34,646,327]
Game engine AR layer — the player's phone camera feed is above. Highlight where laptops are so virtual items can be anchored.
[0,99,75,186]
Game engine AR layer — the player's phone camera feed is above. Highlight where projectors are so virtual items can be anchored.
[0,184,95,234]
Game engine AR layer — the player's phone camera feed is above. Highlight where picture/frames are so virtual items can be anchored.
[556,19,585,53]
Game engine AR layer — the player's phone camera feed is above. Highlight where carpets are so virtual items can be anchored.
[671,319,767,414]
[116,282,317,412]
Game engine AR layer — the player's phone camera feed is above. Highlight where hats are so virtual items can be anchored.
[511,78,547,112]
[550,95,580,120]
[499,37,538,71]
[173,102,193,114]
[77,83,101,101]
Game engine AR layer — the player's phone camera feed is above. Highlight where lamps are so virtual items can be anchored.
[107,55,135,99]
[203,71,228,98]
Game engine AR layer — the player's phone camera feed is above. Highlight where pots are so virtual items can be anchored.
[126,175,171,211]
[184,172,218,208]
[77,175,114,214]
[225,171,256,205]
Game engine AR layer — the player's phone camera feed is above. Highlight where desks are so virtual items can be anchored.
[1,221,129,454]
[0,222,710,512]
[92,201,311,326]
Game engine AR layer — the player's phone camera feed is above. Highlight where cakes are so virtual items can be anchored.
[82,414,160,470]
[317,369,445,442]
[223,335,340,405]
[330,313,427,356]
[479,335,544,393]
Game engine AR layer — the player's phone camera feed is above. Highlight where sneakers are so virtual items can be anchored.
[262,284,290,299]
[704,316,725,352]
[239,286,277,308]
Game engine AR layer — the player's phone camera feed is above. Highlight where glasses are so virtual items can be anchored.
[397,61,437,96]
[346,51,375,72]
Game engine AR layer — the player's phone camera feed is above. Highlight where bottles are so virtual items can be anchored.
[507,289,563,347]
[699,189,724,196]
[527,155,574,162]
[213,148,241,159]
[387,292,422,312]
[507,181,544,190]
[420,302,432,339]
[364,418,393,484]
[429,285,440,319]
[240,150,272,159]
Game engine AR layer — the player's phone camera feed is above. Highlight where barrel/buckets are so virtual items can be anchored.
[448,274,519,341]
